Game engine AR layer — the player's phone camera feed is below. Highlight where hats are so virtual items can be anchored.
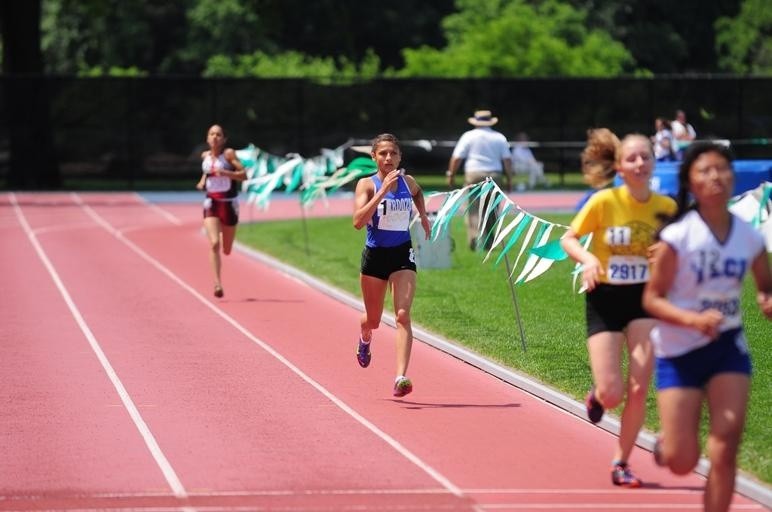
[467,111,497,126]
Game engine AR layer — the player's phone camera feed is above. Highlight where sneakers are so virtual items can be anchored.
[356,330,372,367]
[213,286,222,298]
[586,386,604,424]
[653,434,666,467]
[392,377,412,397]
[611,464,643,486]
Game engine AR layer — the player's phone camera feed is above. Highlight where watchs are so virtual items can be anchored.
[447,171,454,175]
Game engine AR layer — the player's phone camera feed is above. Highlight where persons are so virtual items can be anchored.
[562,128,679,488]
[197,125,247,295]
[650,117,672,159]
[644,141,772,511]
[667,110,697,160]
[354,134,431,397]
[446,110,514,250]
